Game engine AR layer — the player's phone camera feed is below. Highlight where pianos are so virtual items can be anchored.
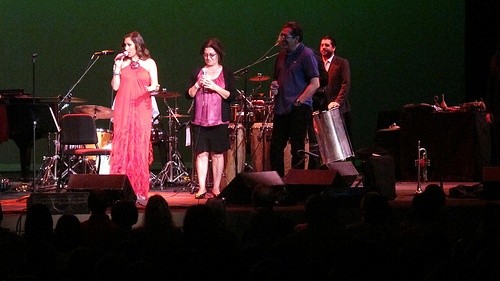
[0,88,60,183]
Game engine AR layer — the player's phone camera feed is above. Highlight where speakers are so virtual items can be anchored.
[66,173,137,212]
[216,170,285,207]
[437,113,491,181]
[363,156,397,201]
[376,111,436,179]
[282,169,350,205]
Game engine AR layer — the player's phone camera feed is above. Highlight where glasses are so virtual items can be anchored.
[281,35,293,39]
[203,53,217,57]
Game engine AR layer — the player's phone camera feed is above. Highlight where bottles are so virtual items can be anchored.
[389,123,396,128]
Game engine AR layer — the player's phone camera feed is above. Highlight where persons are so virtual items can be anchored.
[109,31,159,204]
[268,22,352,205]
[0,188,500,281]
[186,39,236,199]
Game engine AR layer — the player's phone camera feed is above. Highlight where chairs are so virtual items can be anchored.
[61,113,111,179]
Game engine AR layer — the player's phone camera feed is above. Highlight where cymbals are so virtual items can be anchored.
[158,113,190,118]
[57,97,87,103]
[248,75,272,82]
[73,105,115,119]
[380,124,401,132]
[154,91,182,98]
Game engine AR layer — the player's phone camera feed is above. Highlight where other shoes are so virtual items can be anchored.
[211,190,221,199]
[195,191,207,199]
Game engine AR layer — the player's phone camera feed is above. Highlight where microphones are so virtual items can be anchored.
[94,50,114,55]
[202,68,208,93]
[114,51,129,62]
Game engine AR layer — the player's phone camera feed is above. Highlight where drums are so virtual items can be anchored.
[216,105,357,194]
[230,103,266,121]
[78,128,114,160]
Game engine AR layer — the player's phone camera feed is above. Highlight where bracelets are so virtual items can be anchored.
[295,99,301,104]
[113,70,120,75]
[195,82,201,88]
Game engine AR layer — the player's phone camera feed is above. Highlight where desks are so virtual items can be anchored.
[373,108,490,183]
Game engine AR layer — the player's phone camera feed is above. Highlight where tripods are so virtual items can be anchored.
[151,98,190,191]
